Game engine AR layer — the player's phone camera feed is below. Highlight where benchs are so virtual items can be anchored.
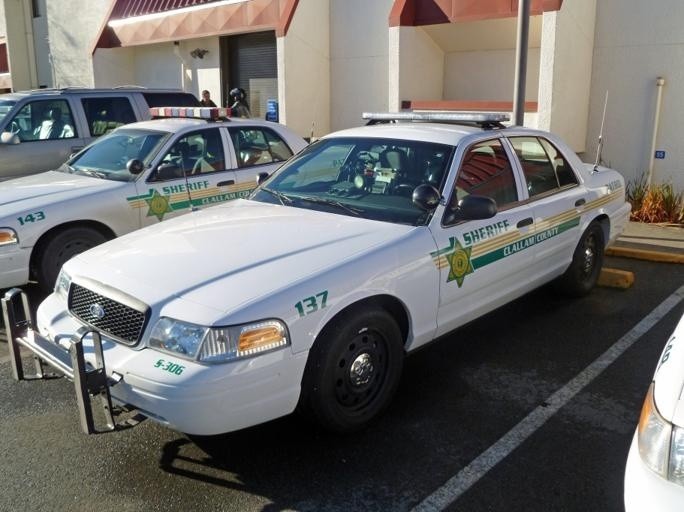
[240,148,277,166]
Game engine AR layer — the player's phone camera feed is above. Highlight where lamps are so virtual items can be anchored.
[191,47,207,60]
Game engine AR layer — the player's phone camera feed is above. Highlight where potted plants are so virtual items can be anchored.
[618,171,684,239]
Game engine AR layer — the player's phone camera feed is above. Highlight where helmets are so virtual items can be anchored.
[230,88,246,100]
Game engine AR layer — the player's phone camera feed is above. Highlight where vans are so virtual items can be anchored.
[1,84,203,181]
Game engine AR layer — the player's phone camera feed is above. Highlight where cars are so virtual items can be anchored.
[1,110,634,437]
[1,104,313,297]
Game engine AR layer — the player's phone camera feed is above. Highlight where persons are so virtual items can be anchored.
[199,89,218,107]
[227,87,252,118]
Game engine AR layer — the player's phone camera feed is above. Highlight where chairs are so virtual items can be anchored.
[38,106,62,141]
[165,142,191,177]
[453,148,497,204]
[374,152,405,196]
[191,136,222,174]
